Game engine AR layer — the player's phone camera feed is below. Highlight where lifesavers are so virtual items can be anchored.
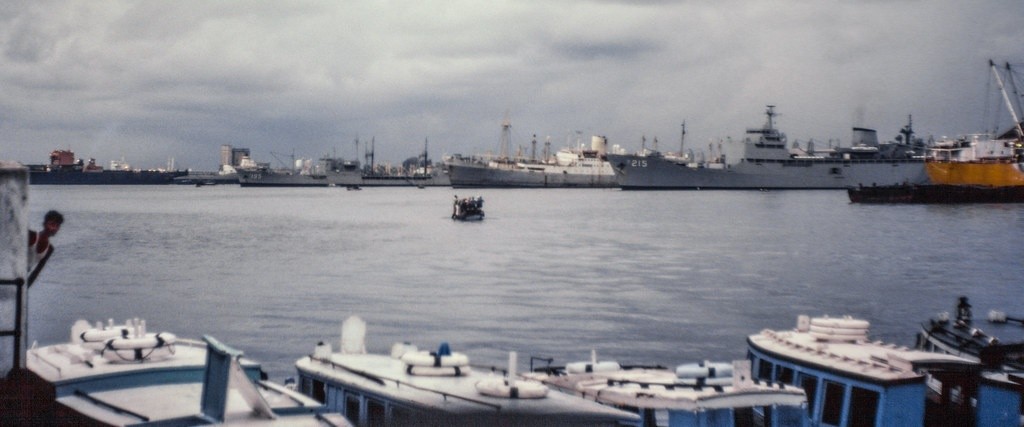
[565,360,619,374]
[403,350,472,379]
[676,360,734,386]
[476,374,552,400]
[105,333,178,351]
[82,324,133,344]
[807,316,872,341]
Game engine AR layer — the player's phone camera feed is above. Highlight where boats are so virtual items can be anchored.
[924,58,1024,188]
[173,152,271,184]
[516,346,813,427]
[602,105,934,188]
[25,149,187,185]
[444,121,687,191]
[746,312,1024,426]
[916,294,1024,380]
[451,195,486,221]
[233,155,435,187]
[25,314,355,427]
[286,315,641,427]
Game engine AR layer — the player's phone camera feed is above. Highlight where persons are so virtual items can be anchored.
[28,209,66,288]
